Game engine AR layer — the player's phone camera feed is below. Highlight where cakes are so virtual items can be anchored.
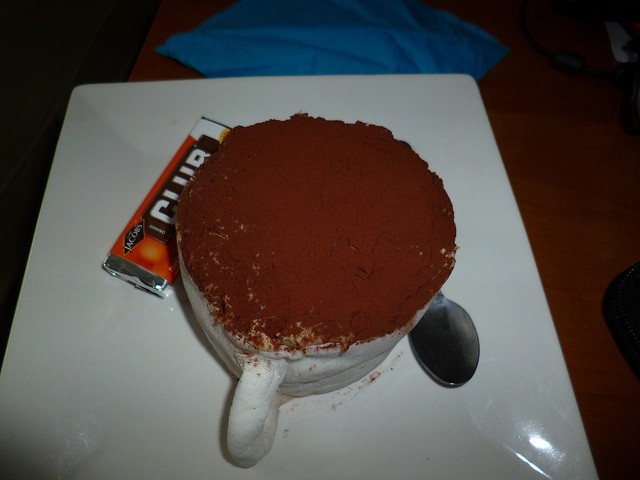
[175,112,458,469]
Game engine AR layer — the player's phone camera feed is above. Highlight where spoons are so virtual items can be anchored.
[407,290,480,388]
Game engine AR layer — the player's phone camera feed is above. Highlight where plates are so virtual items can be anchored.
[0,74,601,479]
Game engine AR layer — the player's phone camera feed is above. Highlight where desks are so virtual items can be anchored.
[125,2,639,480]
